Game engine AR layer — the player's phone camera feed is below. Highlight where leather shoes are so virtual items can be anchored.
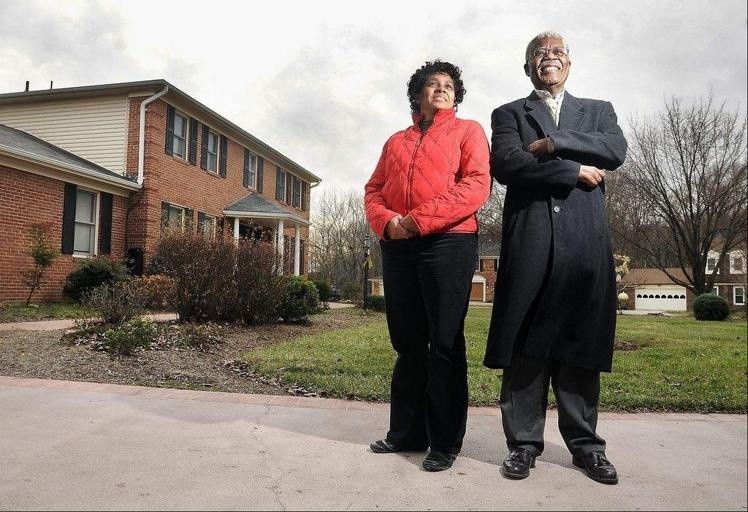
[501,445,537,481]
[571,449,618,487]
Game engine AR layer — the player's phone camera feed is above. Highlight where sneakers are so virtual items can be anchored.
[421,448,459,473]
[368,437,428,454]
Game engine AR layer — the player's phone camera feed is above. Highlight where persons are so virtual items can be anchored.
[488,31,630,485]
[362,59,496,473]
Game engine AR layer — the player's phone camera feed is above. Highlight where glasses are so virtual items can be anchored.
[525,46,567,62]
